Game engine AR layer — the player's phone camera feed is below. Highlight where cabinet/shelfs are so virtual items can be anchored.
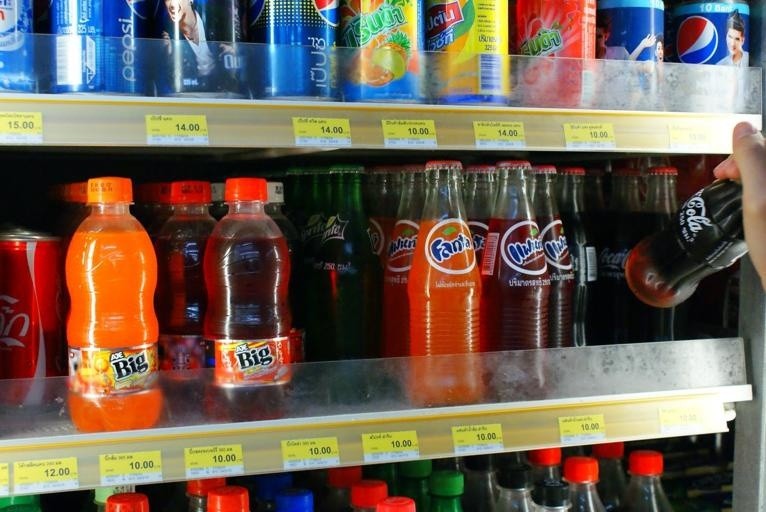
[0,34,766,512]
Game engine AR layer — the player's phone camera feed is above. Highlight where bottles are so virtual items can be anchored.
[1,155,679,433]
[624,179,748,309]
[1,441,673,511]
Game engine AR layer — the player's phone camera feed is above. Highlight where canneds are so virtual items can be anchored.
[1,0,39,94]
[151,2,251,99]
[509,1,597,109]
[0,229,68,417]
[338,0,425,104]
[43,0,145,95]
[596,0,665,111]
[251,0,338,103]
[669,1,749,114]
[425,1,508,105]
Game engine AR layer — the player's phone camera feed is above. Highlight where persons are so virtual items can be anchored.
[158,1,234,93]
[596,8,629,61]
[628,32,664,62]
[715,9,749,67]
[711,121,766,296]
